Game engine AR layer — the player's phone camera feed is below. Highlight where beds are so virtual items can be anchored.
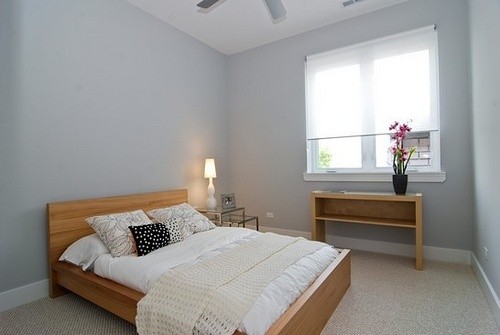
[46,189,351,335]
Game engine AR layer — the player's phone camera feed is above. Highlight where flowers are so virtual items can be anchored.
[388,121,417,174]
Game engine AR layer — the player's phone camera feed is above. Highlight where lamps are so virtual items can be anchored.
[203,158,218,208]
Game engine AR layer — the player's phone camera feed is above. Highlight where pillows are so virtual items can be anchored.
[162,217,183,244]
[84,209,153,258]
[59,233,110,272]
[145,202,217,239]
[128,222,171,257]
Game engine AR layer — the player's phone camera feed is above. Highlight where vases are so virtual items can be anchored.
[392,174,408,195]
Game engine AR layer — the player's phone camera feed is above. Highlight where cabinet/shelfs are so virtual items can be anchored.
[312,190,427,272]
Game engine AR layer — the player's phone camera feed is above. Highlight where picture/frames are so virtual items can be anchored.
[221,193,236,209]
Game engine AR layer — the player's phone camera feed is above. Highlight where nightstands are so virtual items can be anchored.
[195,206,259,232]
